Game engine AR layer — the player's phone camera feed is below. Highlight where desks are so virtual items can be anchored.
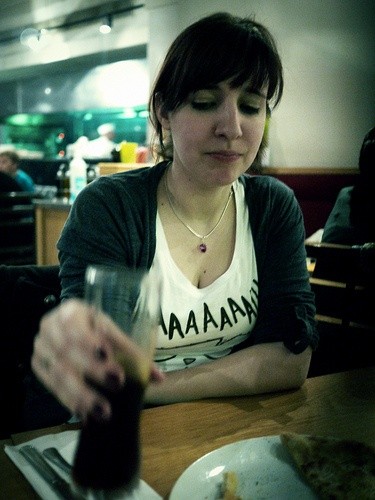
[1,368,374,500]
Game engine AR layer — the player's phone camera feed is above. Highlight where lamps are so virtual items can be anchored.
[99,14,111,35]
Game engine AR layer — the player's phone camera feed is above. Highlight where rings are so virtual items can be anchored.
[39,351,57,371]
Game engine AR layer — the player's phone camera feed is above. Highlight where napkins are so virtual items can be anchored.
[4,429,164,500]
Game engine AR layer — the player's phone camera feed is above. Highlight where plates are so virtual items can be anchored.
[168,434,346,500]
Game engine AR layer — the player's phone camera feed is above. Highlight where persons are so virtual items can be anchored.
[0,151,36,223]
[32,12,316,424]
[314,127,375,284]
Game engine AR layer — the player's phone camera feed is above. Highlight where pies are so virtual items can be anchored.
[279,432,375,500]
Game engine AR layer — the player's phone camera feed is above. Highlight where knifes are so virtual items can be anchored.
[19,444,83,500]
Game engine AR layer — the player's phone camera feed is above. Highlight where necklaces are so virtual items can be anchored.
[165,159,234,254]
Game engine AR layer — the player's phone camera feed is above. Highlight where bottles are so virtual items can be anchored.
[69,151,87,203]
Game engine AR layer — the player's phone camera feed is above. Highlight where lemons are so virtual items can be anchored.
[111,348,150,385]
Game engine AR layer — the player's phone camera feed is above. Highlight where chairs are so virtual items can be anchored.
[0,265,72,438]
[304,243,375,379]
[0,191,40,267]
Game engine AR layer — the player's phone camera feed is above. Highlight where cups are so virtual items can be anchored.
[68,263,162,500]
[121,142,137,164]
